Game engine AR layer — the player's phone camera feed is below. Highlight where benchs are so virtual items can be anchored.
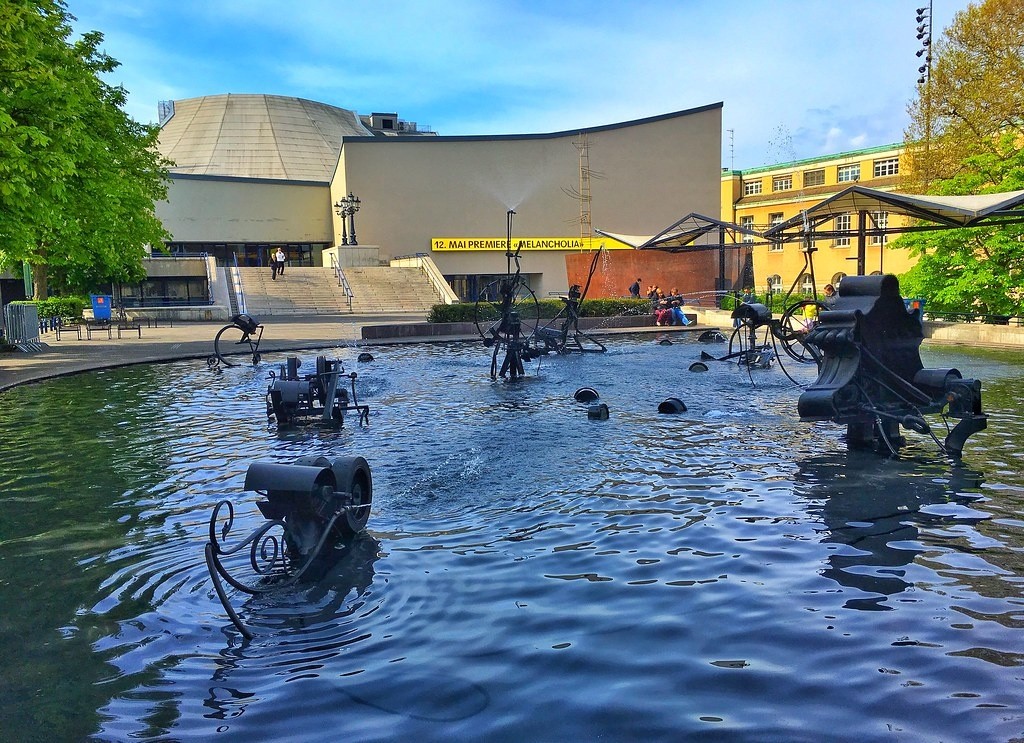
[54,325,82,341]
[86,321,111,340]
[154,317,173,328]
[132,317,151,328]
[117,323,141,339]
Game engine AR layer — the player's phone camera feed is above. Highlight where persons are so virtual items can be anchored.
[647,285,672,326]
[268,253,278,281]
[276,248,286,275]
[802,297,816,333]
[667,288,693,326]
[629,278,641,299]
[733,286,754,329]
[818,284,839,312]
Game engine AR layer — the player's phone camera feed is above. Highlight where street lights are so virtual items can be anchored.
[345,191,361,245]
[335,197,351,246]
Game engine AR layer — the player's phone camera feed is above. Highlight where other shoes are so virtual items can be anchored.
[656,322,661,326]
[665,323,668,325]
[672,322,677,325]
[686,320,695,326]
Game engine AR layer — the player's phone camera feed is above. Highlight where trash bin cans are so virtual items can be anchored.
[902,298,927,331]
[90,295,113,321]
